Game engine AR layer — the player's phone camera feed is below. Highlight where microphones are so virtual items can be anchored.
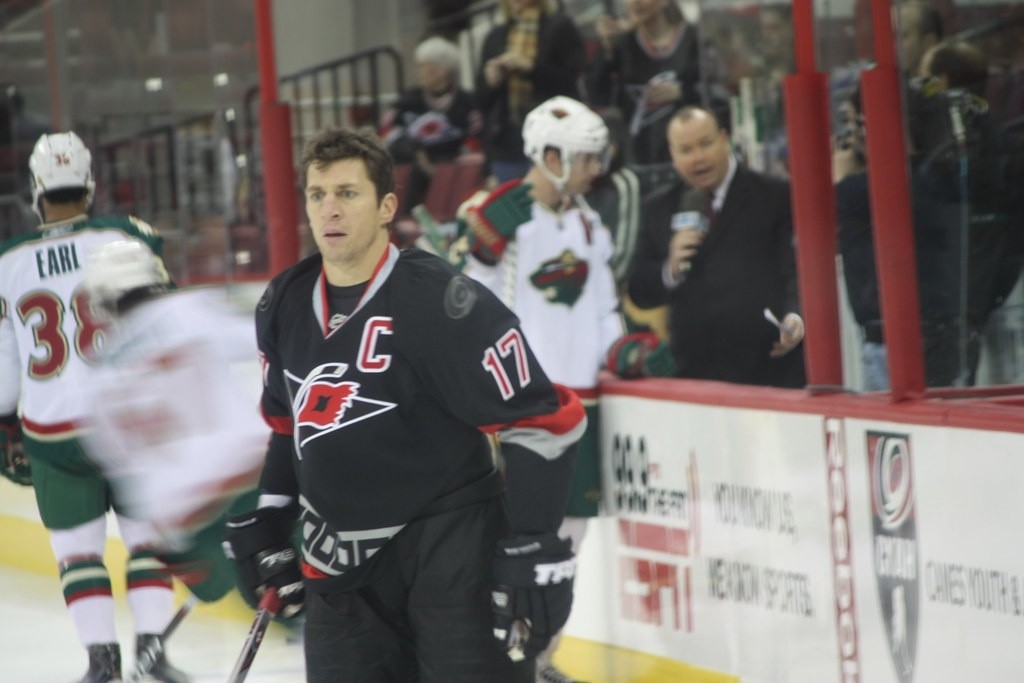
[671,189,710,270]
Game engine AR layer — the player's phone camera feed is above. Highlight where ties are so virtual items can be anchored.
[704,193,717,238]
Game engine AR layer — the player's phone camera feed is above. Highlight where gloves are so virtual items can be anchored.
[222,507,305,622]
[464,175,537,267]
[489,532,576,663]
[607,333,676,380]
[0,411,33,485]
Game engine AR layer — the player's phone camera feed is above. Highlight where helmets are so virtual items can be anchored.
[523,96,609,185]
[28,131,96,223]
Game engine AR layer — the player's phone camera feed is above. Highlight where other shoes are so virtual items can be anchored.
[136,634,190,683]
[76,643,123,683]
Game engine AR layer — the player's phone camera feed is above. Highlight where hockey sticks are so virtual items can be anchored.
[228,588,284,683]
[112,589,199,683]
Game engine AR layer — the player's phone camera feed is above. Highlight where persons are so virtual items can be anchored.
[1,135,195,682]
[451,95,670,682]
[66,238,307,632]
[354,0,1024,396]
[232,126,592,683]
